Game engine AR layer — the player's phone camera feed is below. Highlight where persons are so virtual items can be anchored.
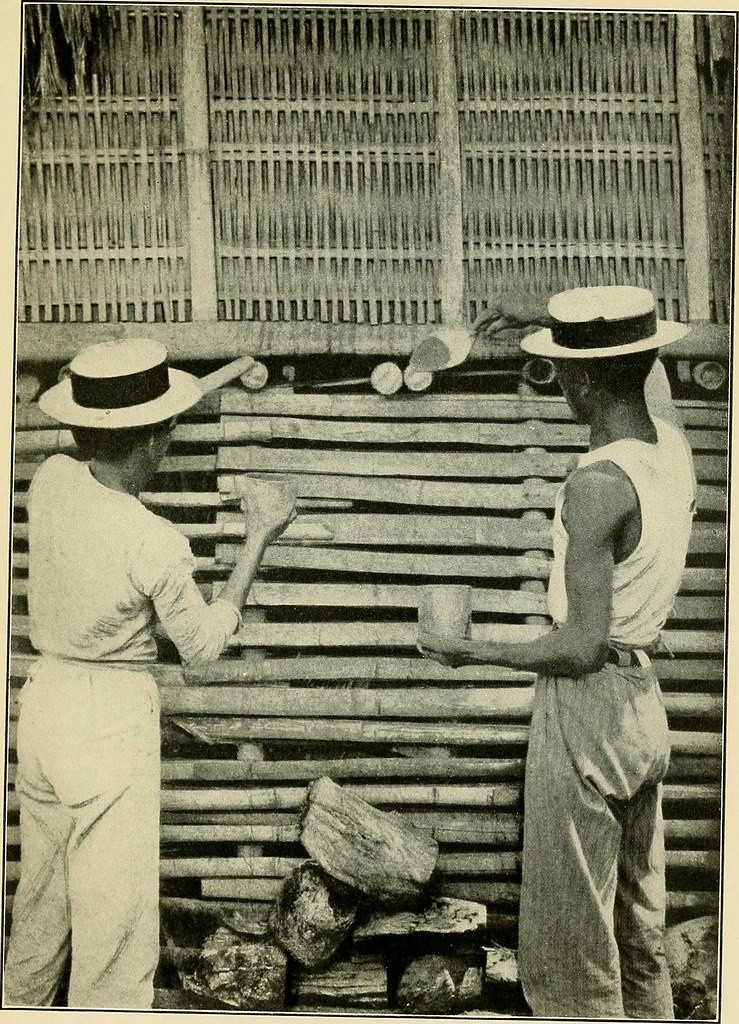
[415,284,697,1020]
[4,339,296,1010]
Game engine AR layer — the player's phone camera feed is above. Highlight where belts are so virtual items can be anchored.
[605,649,652,667]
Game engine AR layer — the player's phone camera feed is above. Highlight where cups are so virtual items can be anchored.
[244,471,298,511]
[418,586,473,644]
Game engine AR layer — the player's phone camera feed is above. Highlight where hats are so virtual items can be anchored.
[38,338,203,429]
[520,286,692,359]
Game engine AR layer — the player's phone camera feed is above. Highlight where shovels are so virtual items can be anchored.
[409,317,493,371]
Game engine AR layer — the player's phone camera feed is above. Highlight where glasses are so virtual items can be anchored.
[151,416,176,434]
